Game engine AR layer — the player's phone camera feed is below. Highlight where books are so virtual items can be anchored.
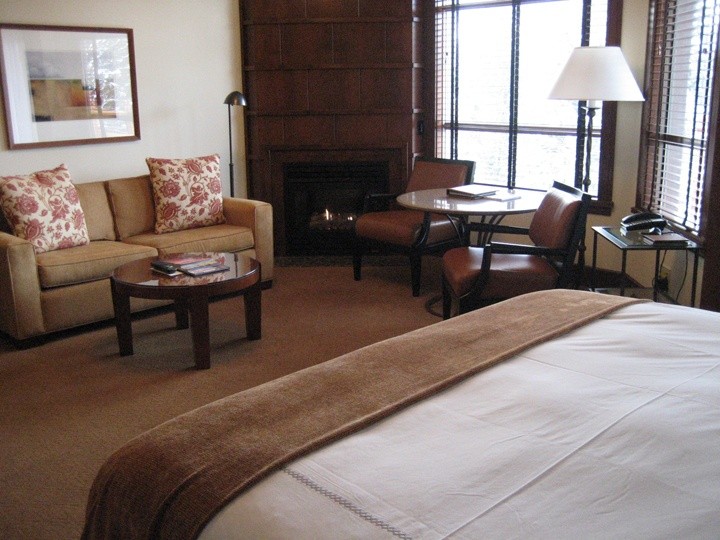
[642,233,689,247]
[163,255,230,278]
[446,183,521,202]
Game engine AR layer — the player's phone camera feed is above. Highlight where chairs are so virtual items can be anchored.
[441,181,591,321]
[352,156,476,297]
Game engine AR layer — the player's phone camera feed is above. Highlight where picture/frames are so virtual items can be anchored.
[0,23,141,149]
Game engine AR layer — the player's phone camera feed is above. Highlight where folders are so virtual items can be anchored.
[446,184,496,199]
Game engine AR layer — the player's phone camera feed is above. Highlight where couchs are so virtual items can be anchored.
[0,176,274,349]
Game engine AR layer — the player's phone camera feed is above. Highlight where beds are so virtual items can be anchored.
[80,288,720,540]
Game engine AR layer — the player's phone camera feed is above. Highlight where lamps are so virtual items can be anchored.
[223,91,247,198]
[547,46,645,290]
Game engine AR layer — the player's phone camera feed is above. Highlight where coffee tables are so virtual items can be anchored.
[110,250,262,370]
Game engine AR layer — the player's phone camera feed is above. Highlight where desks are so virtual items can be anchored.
[591,224,704,307]
[395,186,546,318]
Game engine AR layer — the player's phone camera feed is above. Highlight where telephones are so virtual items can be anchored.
[620,211,668,232]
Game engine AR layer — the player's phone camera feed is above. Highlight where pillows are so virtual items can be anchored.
[145,153,225,234]
[0,163,90,253]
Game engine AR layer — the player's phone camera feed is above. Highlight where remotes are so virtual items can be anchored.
[150,261,179,274]
